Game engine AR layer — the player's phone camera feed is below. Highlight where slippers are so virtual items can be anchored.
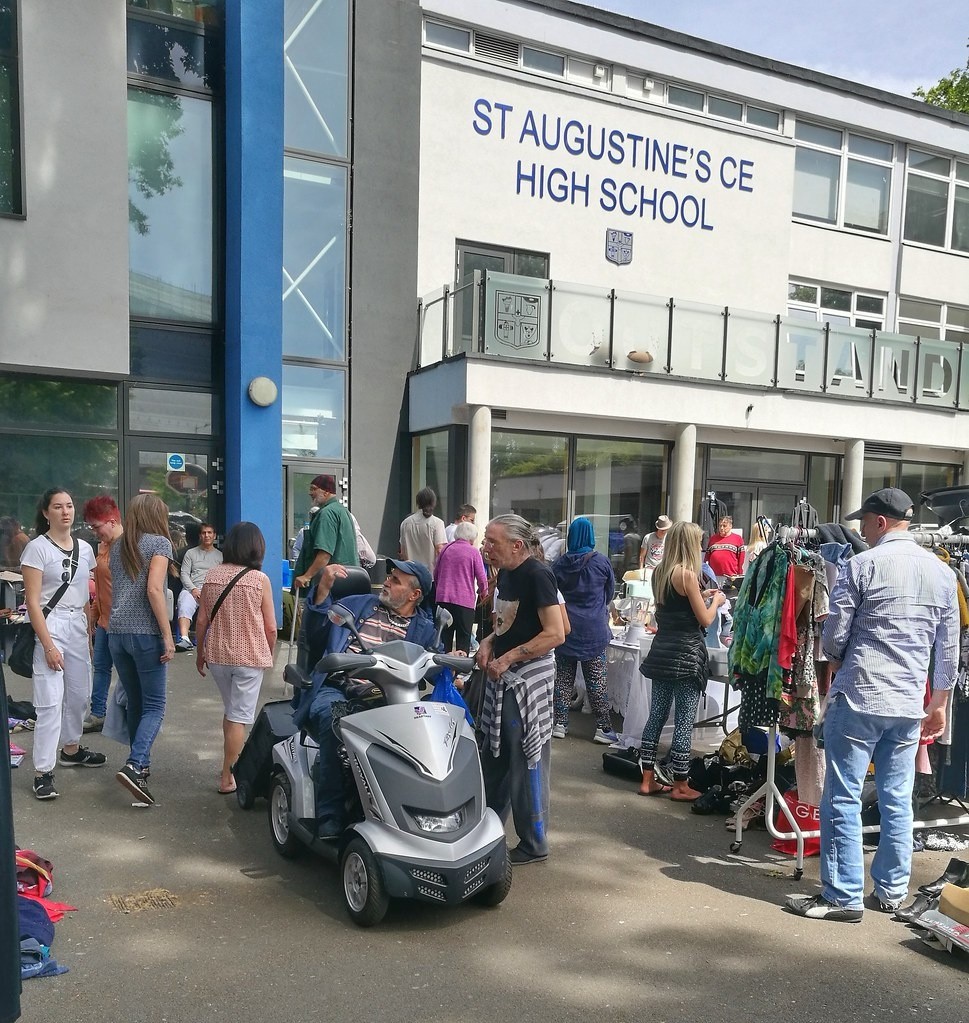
[637,786,673,795]
[671,795,702,802]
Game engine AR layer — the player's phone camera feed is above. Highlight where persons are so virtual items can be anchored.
[0,516,30,575]
[639,515,769,801]
[550,517,620,744]
[83,495,126,733]
[619,517,642,574]
[19,487,106,800]
[399,487,571,865]
[107,493,175,805]
[196,522,278,794]
[289,474,361,600]
[785,487,961,922]
[292,558,466,840]
[175,523,225,652]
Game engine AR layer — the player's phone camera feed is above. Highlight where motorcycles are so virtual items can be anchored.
[265,603,513,928]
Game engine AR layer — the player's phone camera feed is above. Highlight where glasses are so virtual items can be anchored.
[482,539,515,548]
[89,520,110,531]
[310,487,322,492]
[61,558,70,584]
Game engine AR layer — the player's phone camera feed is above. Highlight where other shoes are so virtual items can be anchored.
[84,639,238,794]
[318,815,340,840]
[510,847,549,866]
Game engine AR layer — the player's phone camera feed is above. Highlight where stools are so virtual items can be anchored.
[175,606,211,647]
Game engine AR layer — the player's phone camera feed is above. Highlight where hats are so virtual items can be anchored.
[655,515,674,531]
[311,475,337,494]
[309,506,321,513]
[386,557,432,597]
[844,487,916,521]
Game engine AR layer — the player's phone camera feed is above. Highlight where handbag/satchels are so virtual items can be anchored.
[350,513,377,569]
[431,666,473,726]
[8,621,36,678]
[769,790,820,857]
[601,745,643,783]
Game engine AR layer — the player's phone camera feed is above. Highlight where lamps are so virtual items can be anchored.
[248,377,277,407]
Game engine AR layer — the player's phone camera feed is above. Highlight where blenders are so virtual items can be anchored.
[623,596,650,646]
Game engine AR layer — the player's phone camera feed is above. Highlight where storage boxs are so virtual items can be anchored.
[939,882,969,927]
[707,646,729,677]
[638,635,657,656]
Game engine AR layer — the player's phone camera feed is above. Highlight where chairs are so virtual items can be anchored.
[282,564,373,690]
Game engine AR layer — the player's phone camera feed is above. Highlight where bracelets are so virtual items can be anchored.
[44,646,56,653]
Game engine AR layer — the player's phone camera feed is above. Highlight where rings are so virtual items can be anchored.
[334,572,338,577]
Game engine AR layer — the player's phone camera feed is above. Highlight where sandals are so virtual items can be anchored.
[691,779,769,832]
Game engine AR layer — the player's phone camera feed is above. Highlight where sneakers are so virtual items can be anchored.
[116,763,156,805]
[870,890,902,912]
[553,723,569,739]
[33,772,61,799]
[784,894,864,923]
[59,745,108,768]
[593,728,618,744]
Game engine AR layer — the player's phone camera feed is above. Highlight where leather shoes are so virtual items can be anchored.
[895,895,939,922]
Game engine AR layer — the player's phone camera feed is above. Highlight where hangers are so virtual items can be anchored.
[709,492,717,506]
[759,515,969,564]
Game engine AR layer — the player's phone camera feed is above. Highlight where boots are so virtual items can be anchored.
[917,857,969,896]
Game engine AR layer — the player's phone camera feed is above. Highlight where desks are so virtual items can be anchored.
[609,625,748,737]
